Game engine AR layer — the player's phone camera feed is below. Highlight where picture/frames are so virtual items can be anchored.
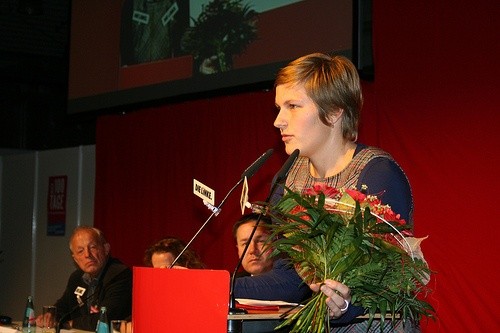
[59,0,374,118]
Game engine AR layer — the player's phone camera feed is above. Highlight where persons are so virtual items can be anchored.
[230,212,279,277]
[116,237,203,333]
[36,225,133,330]
[230,51,414,333]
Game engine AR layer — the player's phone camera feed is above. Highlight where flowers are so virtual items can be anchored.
[251,182,436,333]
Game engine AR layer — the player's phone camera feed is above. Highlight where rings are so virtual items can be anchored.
[341,300,348,311]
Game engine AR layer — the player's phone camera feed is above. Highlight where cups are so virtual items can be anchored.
[42,305,58,333]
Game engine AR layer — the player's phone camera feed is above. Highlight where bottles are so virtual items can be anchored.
[96,307,110,333]
[22,296,36,333]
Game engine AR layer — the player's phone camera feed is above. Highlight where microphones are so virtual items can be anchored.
[56,268,132,333]
[228,148,300,315]
[169,148,274,268]
